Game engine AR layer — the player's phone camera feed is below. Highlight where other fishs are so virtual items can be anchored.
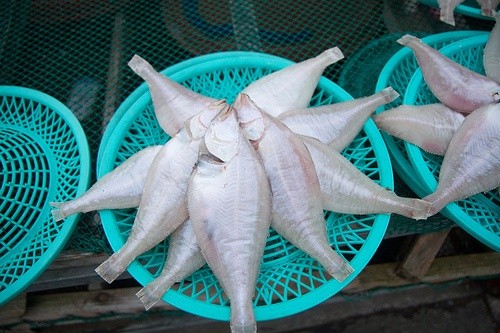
[475,0,499,17]
[294,132,432,222]
[482,8,500,87]
[436,0,464,26]
[236,91,354,284]
[126,53,223,137]
[371,102,466,157]
[95,99,229,285]
[273,85,401,153]
[396,34,500,115]
[50,144,164,222]
[420,102,499,218]
[135,216,208,311]
[238,46,346,119]
[186,106,273,333]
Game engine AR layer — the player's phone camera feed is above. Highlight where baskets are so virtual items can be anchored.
[343,30,431,139]
[96,51,395,321]
[422,0,500,24]
[0,84,92,308]
[402,33,499,252]
[372,29,483,201]
